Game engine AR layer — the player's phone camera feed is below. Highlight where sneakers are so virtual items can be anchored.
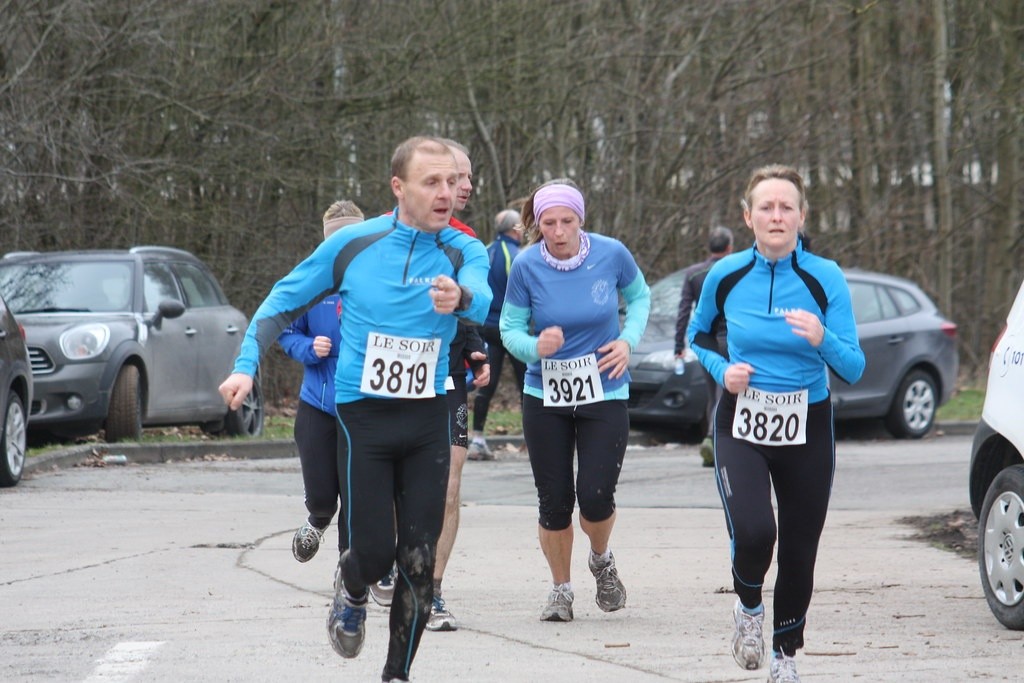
[425,586,457,631]
[767,646,800,683]
[292,518,329,564]
[367,570,397,607]
[730,598,767,671]
[325,549,367,660]
[467,443,495,460]
[539,583,574,622]
[587,550,627,613]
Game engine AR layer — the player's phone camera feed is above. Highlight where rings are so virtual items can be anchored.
[439,301,441,306]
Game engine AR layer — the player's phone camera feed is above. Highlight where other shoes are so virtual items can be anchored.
[699,435,715,468]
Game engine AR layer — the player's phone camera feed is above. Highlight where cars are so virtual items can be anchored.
[0,294,34,489]
[617,261,960,443]
[967,277,1024,632]
[0,245,266,446]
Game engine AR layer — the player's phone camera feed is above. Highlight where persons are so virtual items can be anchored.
[498,178,652,622]
[688,165,866,683]
[674,226,734,467]
[218,134,494,683]
[468,208,526,461]
